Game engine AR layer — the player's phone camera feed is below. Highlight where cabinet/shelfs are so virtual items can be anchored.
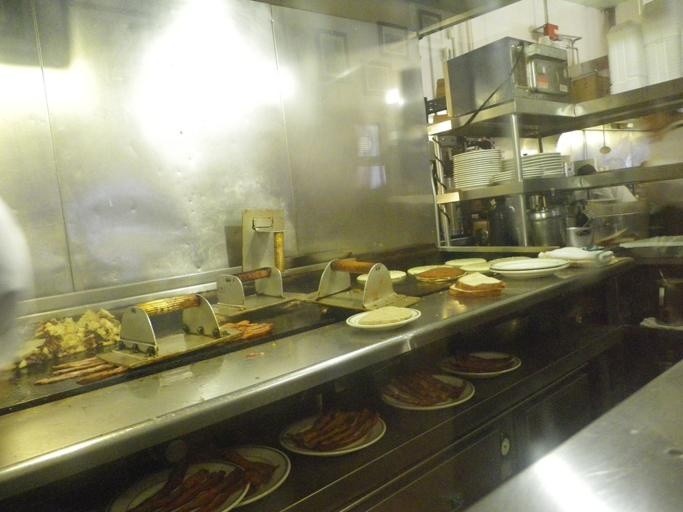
[422,75,681,344]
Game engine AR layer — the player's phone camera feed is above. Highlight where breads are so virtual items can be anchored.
[449,272,505,298]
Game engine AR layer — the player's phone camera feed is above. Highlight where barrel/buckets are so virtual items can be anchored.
[565,227,596,248]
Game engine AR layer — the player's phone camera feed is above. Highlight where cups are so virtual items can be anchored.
[651,277,683,328]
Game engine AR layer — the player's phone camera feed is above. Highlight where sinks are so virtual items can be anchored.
[618,235,683,267]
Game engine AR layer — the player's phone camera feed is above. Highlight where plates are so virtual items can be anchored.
[450,147,564,191]
[436,349,522,379]
[344,307,420,332]
[462,255,567,280]
[379,373,476,413]
[213,445,292,507]
[280,414,387,459]
[107,457,250,511]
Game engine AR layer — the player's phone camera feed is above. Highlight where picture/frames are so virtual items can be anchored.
[310,8,455,98]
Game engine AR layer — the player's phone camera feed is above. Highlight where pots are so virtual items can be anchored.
[585,198,652,239]
[559,226,594,249]
[449,233,473,246]
[527,205,564,247]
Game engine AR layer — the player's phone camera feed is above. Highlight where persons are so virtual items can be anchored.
[631,109,683,308]
[0,199,36,365]
[577,164,637,202]
